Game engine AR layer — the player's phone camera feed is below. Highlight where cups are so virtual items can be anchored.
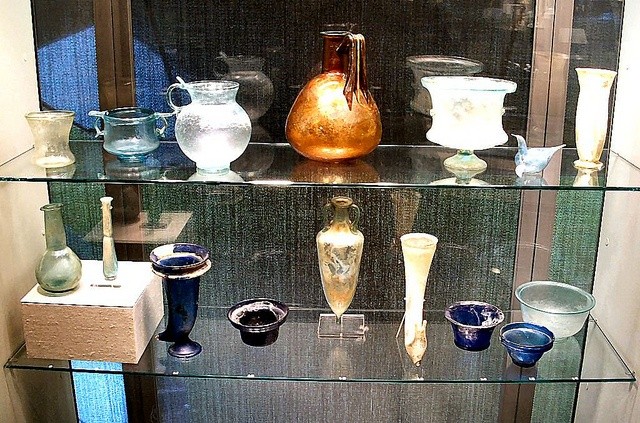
[25,110,76,168]
[444,299,505,351]
[88,106,172,163]
[498,322,556,369]
[225,297,290,347]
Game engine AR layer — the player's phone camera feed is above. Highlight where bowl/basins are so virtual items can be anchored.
[515,280,595,341]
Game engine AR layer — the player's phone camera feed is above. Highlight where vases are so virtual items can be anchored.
[394,233,436,367]
[314,196,369,341]
[284,29,383,162]
[147,242,211,359]
[30,203,81,295]
[98,195,123,285]
[574,67,618,189]
